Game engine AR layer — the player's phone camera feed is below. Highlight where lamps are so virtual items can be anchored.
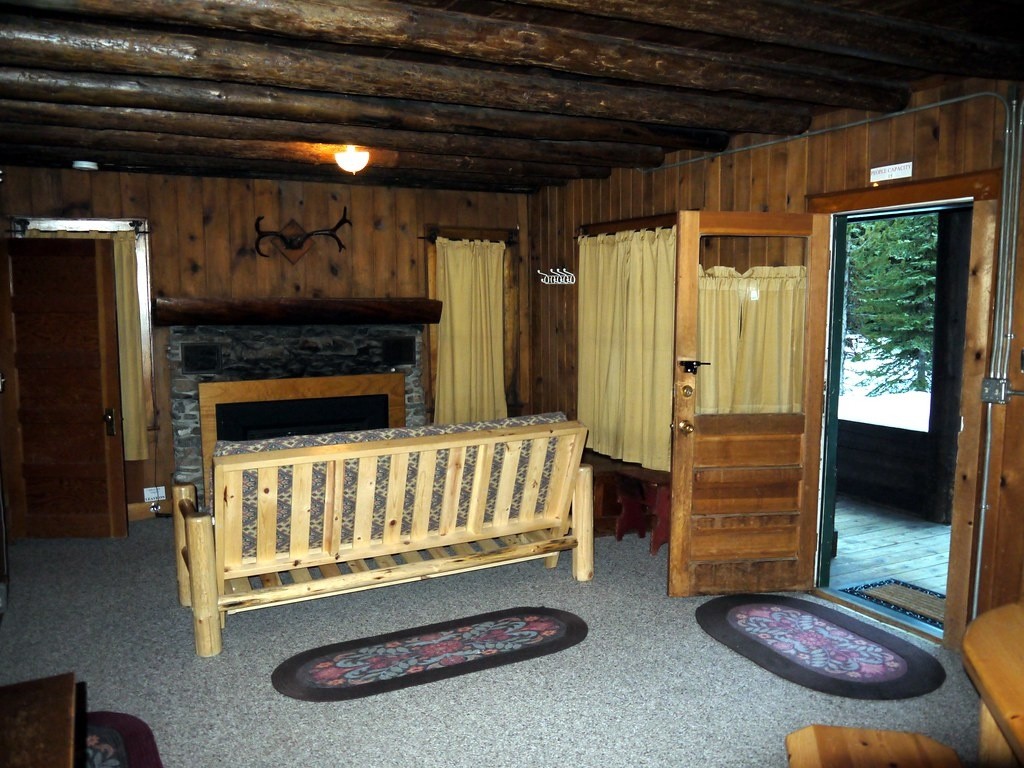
[333,144,370,176]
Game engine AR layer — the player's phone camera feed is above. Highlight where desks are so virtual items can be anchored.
[0,670,79,768]
[961,598,1024,768]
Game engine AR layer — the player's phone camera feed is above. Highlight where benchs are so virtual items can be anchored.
[171,410,593,657]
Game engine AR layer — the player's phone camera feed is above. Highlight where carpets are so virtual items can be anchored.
[272,605,589,702]
[840,578,946,629]
[694,591,946,700]
[78,712,163,768]
